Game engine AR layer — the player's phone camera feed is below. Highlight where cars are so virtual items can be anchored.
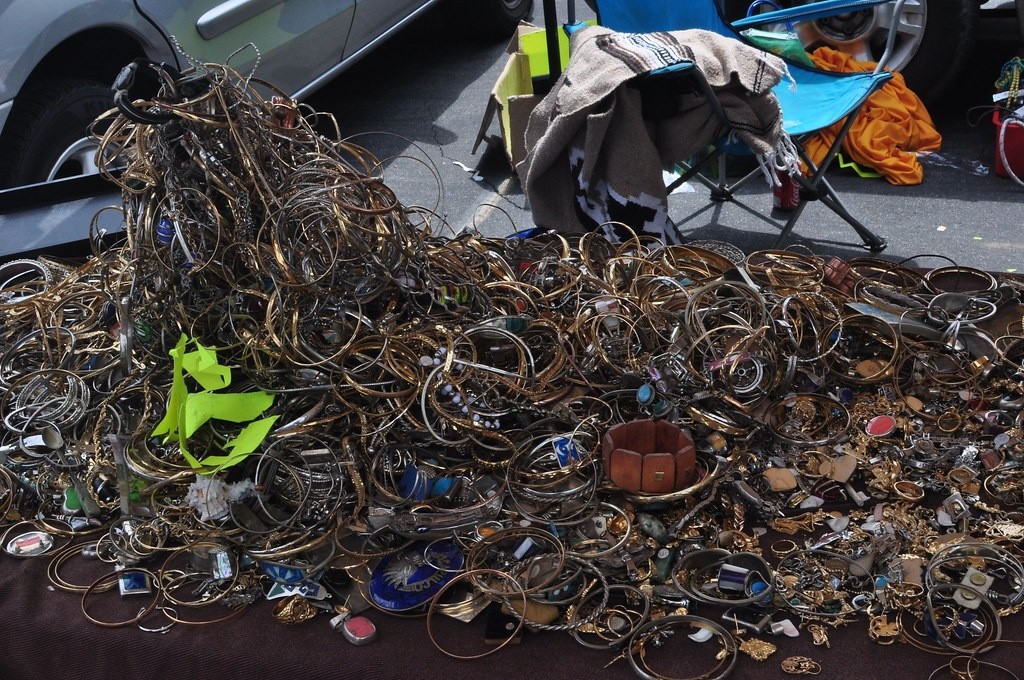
[0,0,536,274]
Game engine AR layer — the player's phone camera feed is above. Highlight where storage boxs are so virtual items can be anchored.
[994,104,1024,177]
[470,19,595,168]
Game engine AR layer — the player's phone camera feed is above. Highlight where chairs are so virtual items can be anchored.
[516,0,904,264]
